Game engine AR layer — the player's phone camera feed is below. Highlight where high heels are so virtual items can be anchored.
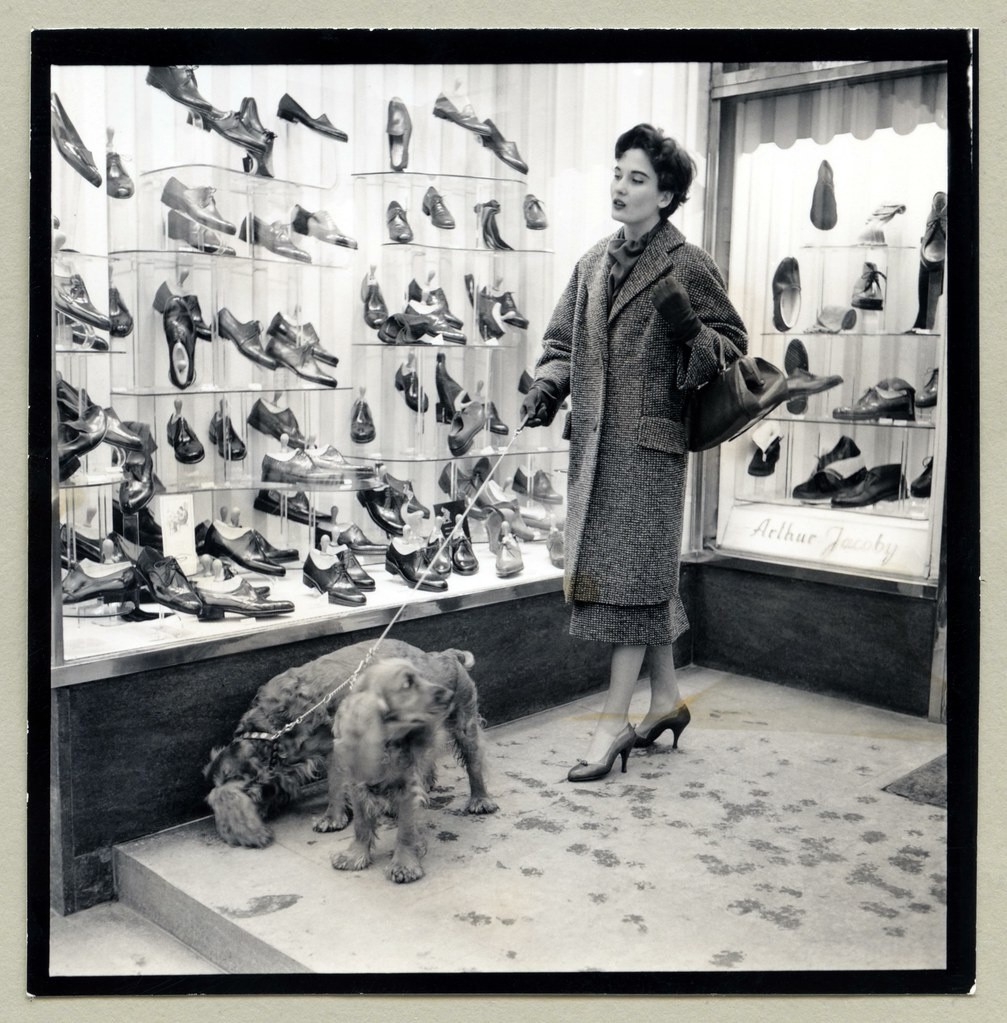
[567,722,638,783]
[634,704,691,749]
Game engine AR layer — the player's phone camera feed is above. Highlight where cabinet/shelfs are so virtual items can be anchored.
[341,170,570,547]
[107,162,385,580]
[50,247,137,619]
[731,243,942,522]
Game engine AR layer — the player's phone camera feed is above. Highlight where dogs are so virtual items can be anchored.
[333,653,498,885]
[203,637,432,849]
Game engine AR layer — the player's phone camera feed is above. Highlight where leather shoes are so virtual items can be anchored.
[51,64,569,622]
[831,463,910,508]
[920,191,947,271]
[792,435,867,500]
[832,385,916,422]
[784,338,809,415]
[915,368,939,408]
[851,262,888,311]
[910,456,933,498]
[786,368,843,397]
[771,257,802,333]
[748,421,785,476]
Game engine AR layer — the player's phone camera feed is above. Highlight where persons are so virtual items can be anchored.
[520,122,749,782]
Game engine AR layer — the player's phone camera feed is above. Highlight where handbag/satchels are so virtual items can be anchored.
[688,333,786,452]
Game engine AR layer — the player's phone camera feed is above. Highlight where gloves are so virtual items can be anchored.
[519,377,562,429]
[649,274,702,343]
